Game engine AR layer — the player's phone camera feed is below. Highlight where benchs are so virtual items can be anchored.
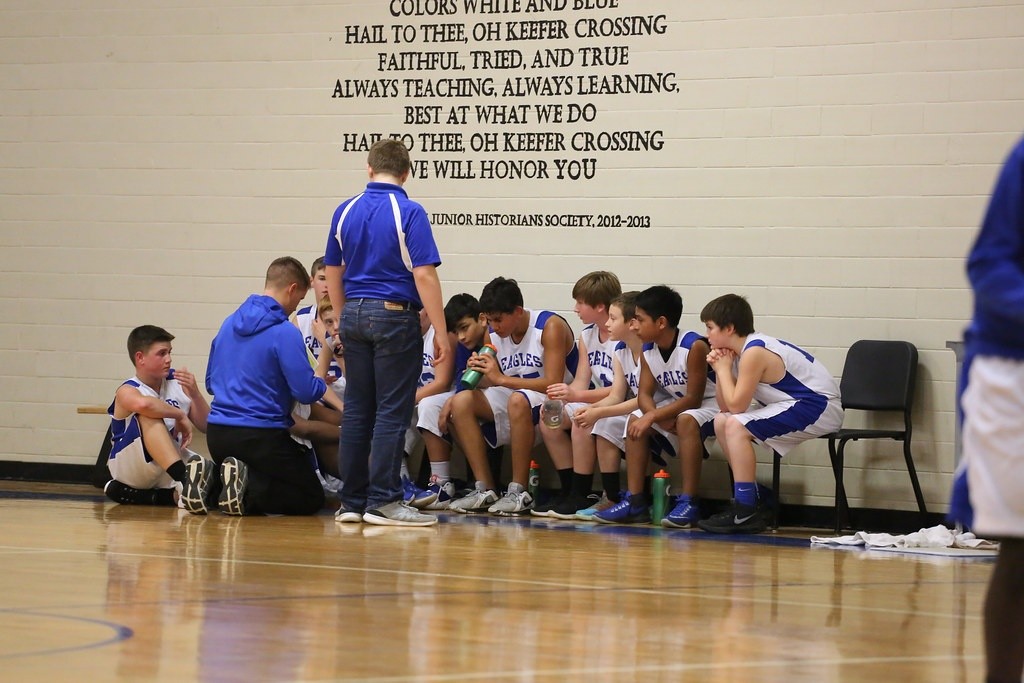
[73,398,114,487]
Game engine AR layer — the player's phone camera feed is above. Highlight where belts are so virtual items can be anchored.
[346,298,420,311]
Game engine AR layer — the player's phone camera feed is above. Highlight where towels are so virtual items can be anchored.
[810,523,1002,552]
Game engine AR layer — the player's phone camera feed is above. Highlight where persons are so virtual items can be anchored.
[181,257,327,515]
[325,140,450,526]
[961,138,1024,682]
[530,272,622,520]
[591,286,720,528]
[450,277,579,516]
[698,294,844,534]
[573,290,671,521]
[289,257,346,493]
[107,325,211,509]
[400,307,458,507]
[411,295,495,510]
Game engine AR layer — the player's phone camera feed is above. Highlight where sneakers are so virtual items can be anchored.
[334,503,363,523]
[487,481,537,517]
[217,456,249,516]
[575,488,619,521]
[182,454,214,515]
[448,481,503,514]
[103,479,158,506]
[592,489,652,524]
[363,501,439,527]
[698,498,767,534]
[424,481,456,509]
[530,496,595,519]
[401,474,424,494]
[660,492,703,529]
[412,481,446,508]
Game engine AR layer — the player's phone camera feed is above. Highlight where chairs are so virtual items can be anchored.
[767,337,928,538]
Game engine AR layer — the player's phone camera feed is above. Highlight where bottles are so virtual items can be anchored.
[460,344,498,389]
[528,460,541,502]
[332,334,344,357]
[652,469,672,525]
[541,393,563,429]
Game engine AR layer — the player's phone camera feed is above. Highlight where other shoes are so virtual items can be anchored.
[402,492,415,505]
